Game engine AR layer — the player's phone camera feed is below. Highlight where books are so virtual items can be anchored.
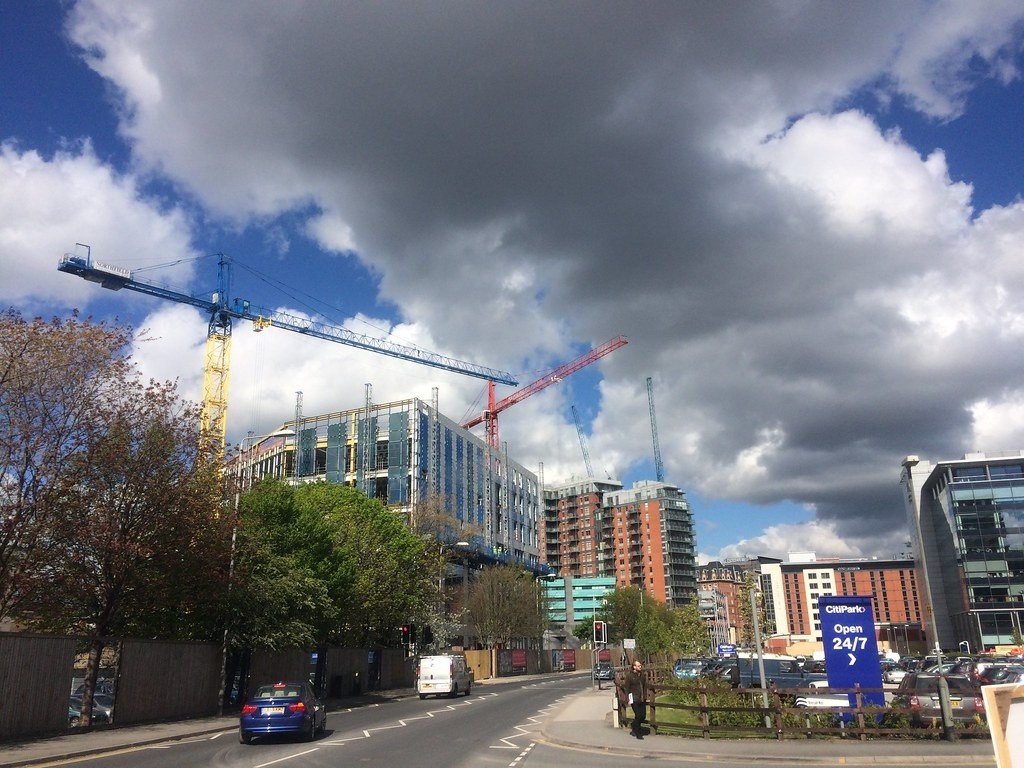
[628,693,634,705]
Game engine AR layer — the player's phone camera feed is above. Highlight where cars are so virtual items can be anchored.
[239,683,327,743]
[592,664,613,680]
[69,679,114,728]
[674,652,827,691]
[879,652,1024,729]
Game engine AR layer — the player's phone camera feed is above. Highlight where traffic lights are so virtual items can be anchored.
[402,625,410,643]
[426,626,433,644]
[593,621,607,645]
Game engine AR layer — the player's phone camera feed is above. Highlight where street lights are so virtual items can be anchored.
[901,455,956,742]
[214,430,298,716]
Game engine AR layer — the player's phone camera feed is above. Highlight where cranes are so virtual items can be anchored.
[55,242,517,540]
[571,403,600,477]
[644,377,664,483]
[461,335,628,569]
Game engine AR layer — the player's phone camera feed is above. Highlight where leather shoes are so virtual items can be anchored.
[636,733,644,740]
[631,723,635,733]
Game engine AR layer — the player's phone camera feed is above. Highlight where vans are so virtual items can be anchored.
[418,655,473,699]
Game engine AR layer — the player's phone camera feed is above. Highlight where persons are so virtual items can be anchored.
[620,653,626,667]
[625,661,647,740]
[714,667,721,673]
